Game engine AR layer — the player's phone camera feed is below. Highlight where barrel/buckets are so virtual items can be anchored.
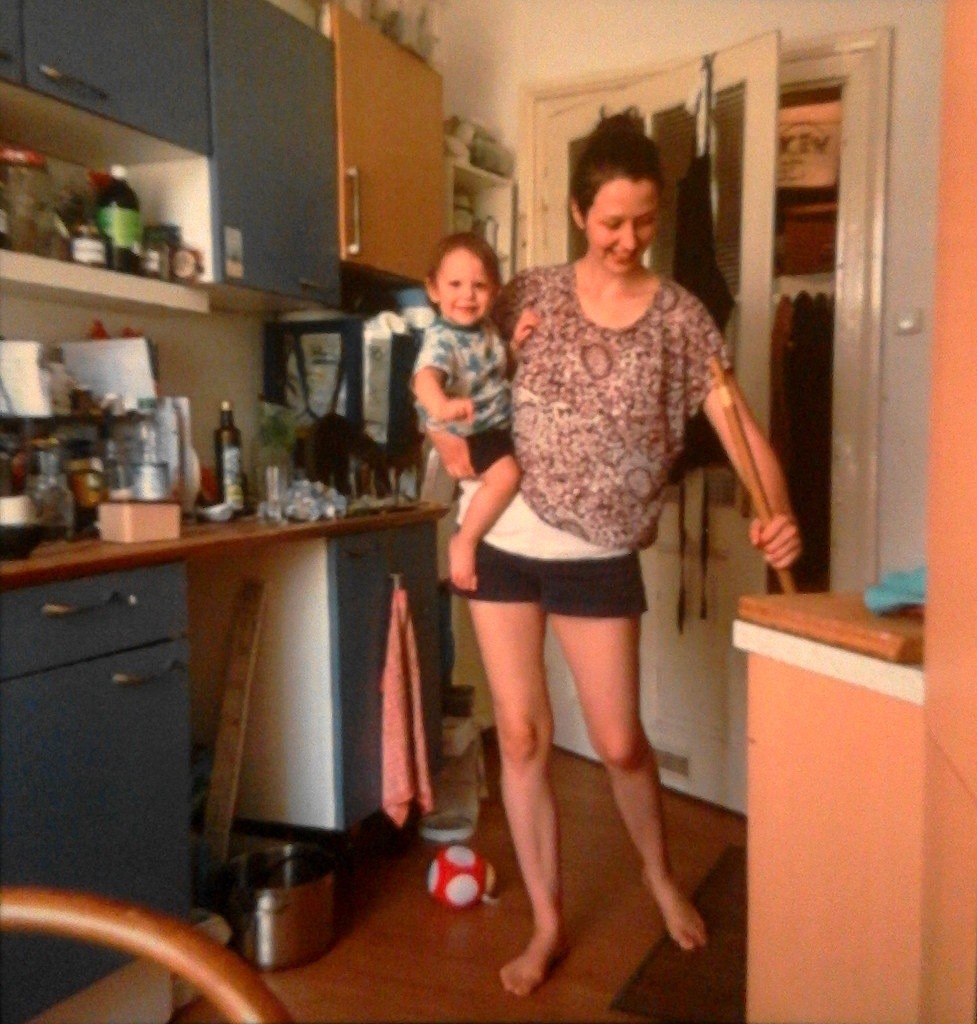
[223,839,342,968]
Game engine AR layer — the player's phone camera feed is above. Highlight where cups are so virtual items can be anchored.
[256,476,347,525]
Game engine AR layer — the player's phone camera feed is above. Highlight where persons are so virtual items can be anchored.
[408,232,540,590]
[426,113,801,997]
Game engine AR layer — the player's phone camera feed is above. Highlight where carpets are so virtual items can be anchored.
[602,841,747,1024]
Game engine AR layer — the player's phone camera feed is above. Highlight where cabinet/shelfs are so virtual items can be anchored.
[0,0,523,325]
[735,0,977,1024]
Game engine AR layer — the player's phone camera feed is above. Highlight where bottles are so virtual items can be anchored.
[0,143,190,283]
[213,398,247,515]
[0,359,169,529]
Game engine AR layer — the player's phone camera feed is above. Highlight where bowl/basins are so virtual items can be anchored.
[0,523,68,559]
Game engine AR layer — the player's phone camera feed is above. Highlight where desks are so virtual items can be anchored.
[0,494,450,1024]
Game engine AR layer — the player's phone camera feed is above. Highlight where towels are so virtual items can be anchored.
[380,586,440,829]
